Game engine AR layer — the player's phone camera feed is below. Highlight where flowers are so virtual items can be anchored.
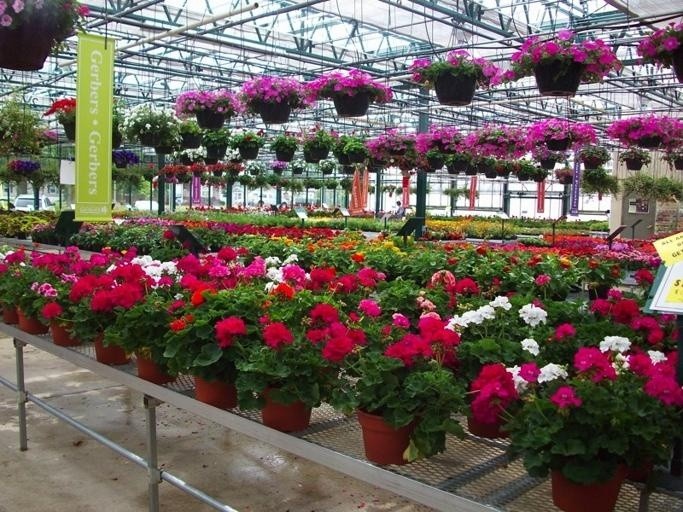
[1,1,88,71]
[1,88,683,346]
[411,49,504,105]
[500,359,658,489]
[306,69,393,118]
[243,74,309,124]
[571,335,683,458]
[163,281,274,377]
[636,19,683,85]
[444,294,554,420]
[503,30,624,97]
[309,297,467,456]
[216,286,355,409]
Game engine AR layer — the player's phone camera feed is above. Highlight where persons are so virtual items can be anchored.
[387,201,404,220]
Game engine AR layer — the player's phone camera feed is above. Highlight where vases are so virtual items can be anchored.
[259,386,312,433]
[194,373,237,409]
[624,451,654,482]
[133,347,178,384]
[93,330,130,365]
[354,408,419,464]
[548,459,629,511]
[466,397,509,437]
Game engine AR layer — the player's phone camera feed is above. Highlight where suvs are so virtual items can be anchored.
[12,192,52,211]
[244,189,289,210]
